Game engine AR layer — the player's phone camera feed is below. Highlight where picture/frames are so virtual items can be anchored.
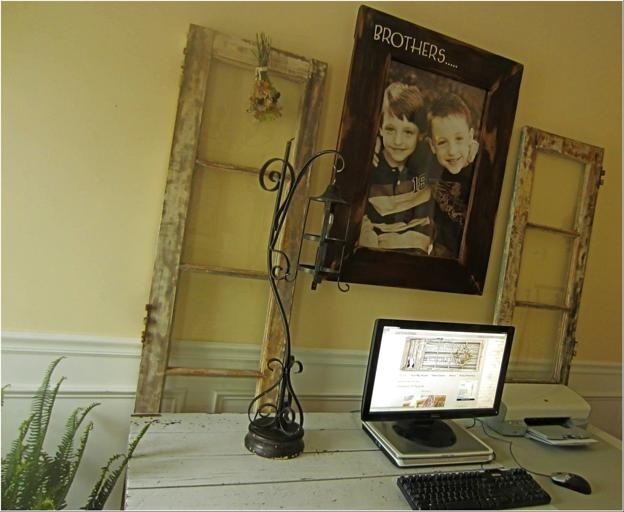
[311,5,525,297]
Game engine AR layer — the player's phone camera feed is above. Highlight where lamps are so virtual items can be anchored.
[243,137,354,461]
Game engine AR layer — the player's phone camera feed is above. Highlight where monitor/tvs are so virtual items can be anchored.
[361,319,515,447]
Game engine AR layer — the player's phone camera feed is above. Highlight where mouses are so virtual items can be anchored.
[551,472,592,495]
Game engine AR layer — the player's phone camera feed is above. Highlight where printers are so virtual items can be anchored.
[487,383,601,447]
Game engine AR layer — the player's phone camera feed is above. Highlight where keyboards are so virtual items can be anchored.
[397,467,552,510]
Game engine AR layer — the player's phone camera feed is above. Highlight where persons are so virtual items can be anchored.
[423,91,497,258]
[357,78,437,258]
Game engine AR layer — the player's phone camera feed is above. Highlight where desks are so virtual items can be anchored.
[120,410,622,510]
